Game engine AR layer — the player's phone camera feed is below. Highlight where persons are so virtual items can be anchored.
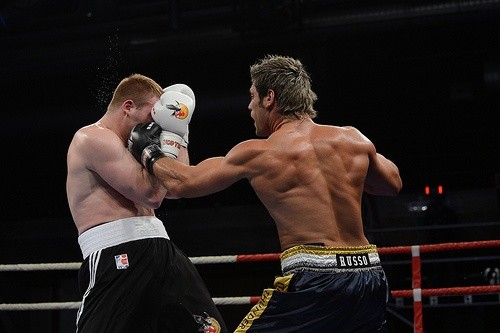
[126,53,404,333]
[65,72,229,332]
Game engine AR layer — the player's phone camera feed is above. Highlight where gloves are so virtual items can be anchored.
[127,121,167,175]
[151,84,197,160]
